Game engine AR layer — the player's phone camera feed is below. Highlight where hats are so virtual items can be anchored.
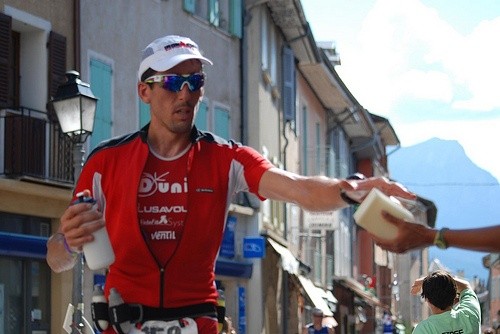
[138,35,213,83]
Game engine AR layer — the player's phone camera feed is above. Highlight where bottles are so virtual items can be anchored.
[72,192,116,271]
[92,284,131,334]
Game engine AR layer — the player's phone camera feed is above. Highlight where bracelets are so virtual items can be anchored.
[340,175,362,205]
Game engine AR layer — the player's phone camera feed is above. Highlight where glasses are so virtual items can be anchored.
[143,73,206,92]
[314,315,322,317]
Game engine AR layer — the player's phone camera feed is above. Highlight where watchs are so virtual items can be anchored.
[433,227,448,250]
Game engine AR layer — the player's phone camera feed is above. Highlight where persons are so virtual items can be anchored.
[46,35,416,334]
[366,209,500,334]
[303,307,333,334]
